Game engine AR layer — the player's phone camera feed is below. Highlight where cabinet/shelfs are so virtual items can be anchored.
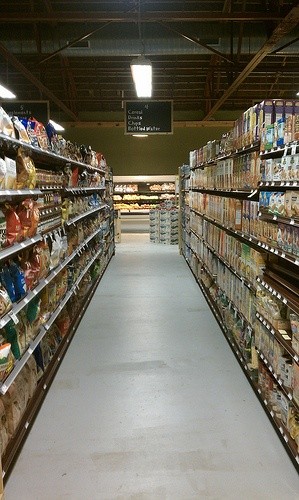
[112,175,181,218]
[178,124,299,479]
[1,132,115,486]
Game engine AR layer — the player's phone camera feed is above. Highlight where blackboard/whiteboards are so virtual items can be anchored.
[123,99,174,135]
[1,100,51,131]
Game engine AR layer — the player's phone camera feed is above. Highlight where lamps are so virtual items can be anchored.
[49,104,67,132]
[0,56,17,98]
[131,46,153,98]
